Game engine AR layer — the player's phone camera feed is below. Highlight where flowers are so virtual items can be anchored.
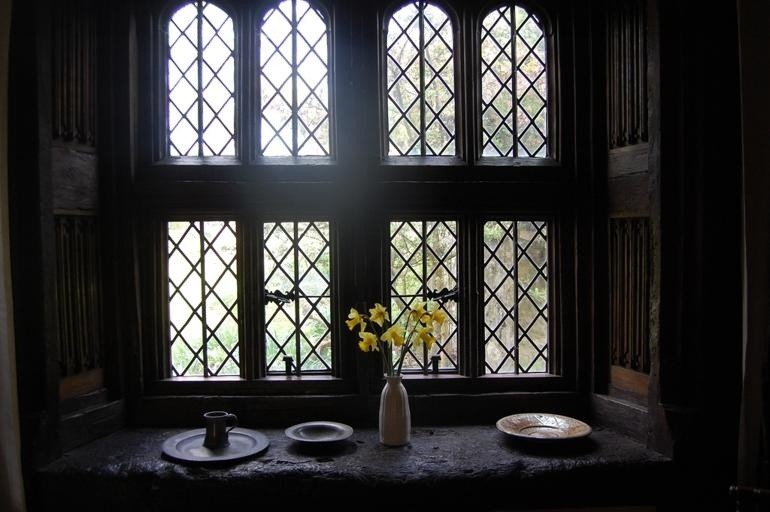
[344,302,444,376]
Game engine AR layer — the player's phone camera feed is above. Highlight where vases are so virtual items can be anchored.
[378,375,412,448]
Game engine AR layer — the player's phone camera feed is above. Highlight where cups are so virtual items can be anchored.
[202,409,238,450]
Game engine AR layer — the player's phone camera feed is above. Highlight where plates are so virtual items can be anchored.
[159,424,271,463]
[493,410,594,444]
[283,421,355,446]
